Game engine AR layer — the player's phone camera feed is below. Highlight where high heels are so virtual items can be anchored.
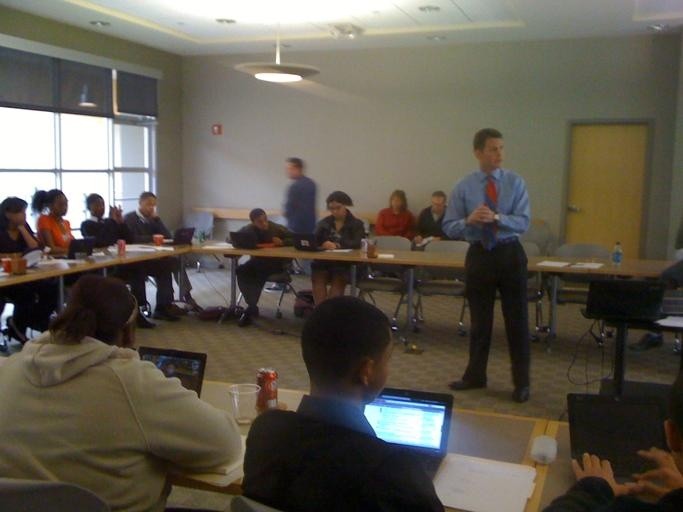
[5,316,31,345]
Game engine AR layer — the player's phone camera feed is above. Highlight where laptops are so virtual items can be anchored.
[293,233,326,251]
[581,281,667,321]
[229,231,263,250]
[164,228,195,245]
[54,236,95,259]
[567,393,671,503]
[138,346,207,398]
[364,388,454,480]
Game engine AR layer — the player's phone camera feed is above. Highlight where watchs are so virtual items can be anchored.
[492,211,500,225]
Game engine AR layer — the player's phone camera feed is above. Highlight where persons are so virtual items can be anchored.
[373,189,416,240]
[160,360,182,380]
[263,157,316,294]
[123,190,189,322]
[414,189,453,244]
[437,127,532,403]
[541,370,681,512]
[307,187,364,308]
[242,294,446,512]
[30,188,78,260]
[0,273,246,511]
[224,208,294,328]
[626,217,682,351]
[80,192,156,330]
[0,196,57,346]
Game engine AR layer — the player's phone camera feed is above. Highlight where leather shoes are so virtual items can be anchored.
[137,304,188,329]
[511,386,529,403]
[447,379,486,390]
[627,333,663,351]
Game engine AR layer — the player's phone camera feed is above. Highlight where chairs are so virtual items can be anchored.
[312,215,376,306]
[235,216,298,318]
[521,221,566,303]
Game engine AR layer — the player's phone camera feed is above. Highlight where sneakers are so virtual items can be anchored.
[237,306,259,327]
[264,284,283,293]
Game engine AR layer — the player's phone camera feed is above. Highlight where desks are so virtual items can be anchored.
[192,209,379,272]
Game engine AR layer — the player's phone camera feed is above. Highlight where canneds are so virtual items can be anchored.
[117,239,126,257]
[256,367,279,414]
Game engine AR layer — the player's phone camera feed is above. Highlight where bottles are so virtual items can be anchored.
[610,240,623,269]
[359,230,371,253]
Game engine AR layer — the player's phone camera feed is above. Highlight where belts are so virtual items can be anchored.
[470,236,519,250]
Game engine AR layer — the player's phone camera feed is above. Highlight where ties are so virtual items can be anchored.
[478,176,498,252]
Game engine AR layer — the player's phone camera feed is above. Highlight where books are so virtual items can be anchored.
[193,434,246,476]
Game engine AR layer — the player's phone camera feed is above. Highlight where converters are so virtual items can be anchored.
[273,328,282,334]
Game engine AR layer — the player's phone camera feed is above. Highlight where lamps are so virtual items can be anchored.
[234,36,320,83]
[77,85,99,108]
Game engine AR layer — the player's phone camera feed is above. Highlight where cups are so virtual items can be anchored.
[152,235,163,248]
[226,383,261,425]
[365,239,378,259]
[1,258,12,274]
